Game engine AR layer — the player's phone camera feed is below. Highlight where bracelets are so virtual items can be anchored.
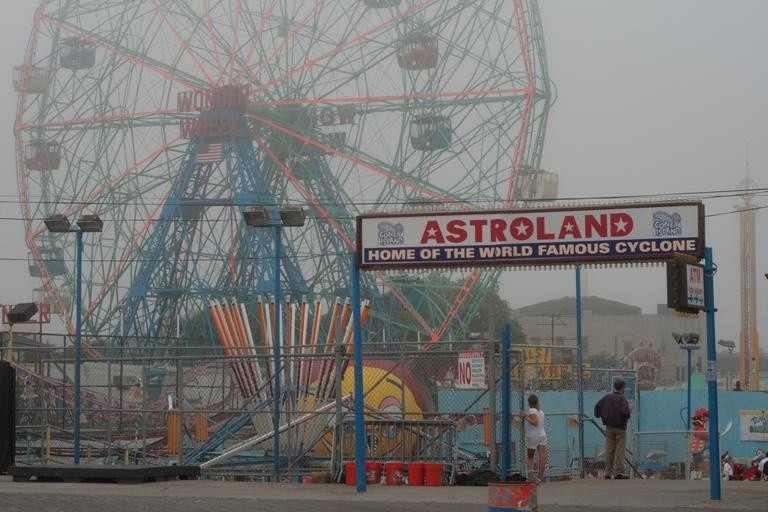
[523,415,528,418]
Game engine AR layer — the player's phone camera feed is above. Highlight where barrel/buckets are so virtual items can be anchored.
[407,462,423,485]
[487,480,539,511]
[343,462,357,484]
[385,462,404,485]
[365,462,381,484]
[424,462,444,486]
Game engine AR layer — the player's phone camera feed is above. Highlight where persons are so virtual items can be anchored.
[691,407,710,481]
[520,395,547,484]
[595,380,631,479]
[721,449,768,481]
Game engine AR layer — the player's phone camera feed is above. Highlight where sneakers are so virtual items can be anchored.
[604,474,612,479]
[614,474,630,479]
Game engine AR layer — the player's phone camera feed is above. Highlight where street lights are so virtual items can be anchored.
[243,202,312,481]
[673,329,702,432]
[717,336,738,388]
[0,302,38,366]
[44,212,102,467]
